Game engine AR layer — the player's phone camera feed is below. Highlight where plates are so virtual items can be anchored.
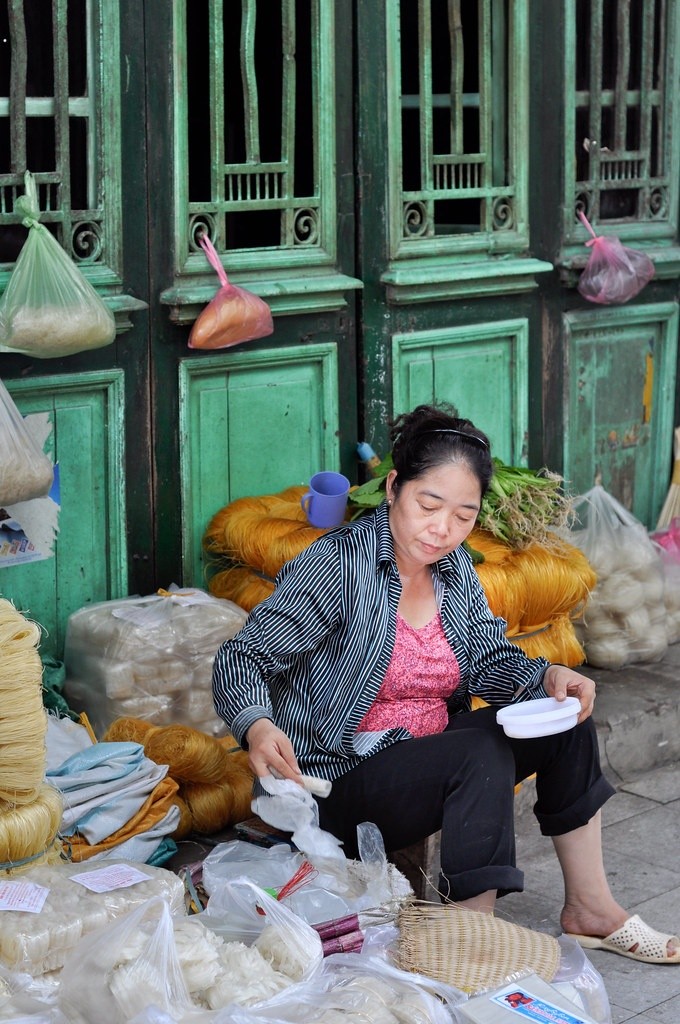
[495,694,580,738]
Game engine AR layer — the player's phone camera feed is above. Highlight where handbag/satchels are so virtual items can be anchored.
[60,839,468,1024]
[0,170,118,359]
[548,486,680,670]
[576,208,656,306]
[0,380,55,508]
[188,233,275,351]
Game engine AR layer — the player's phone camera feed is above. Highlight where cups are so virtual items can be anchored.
[300,471,350,530]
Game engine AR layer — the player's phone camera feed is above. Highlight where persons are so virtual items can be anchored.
[211,402,680,966]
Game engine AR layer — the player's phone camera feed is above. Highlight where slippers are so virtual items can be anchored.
[563,915,680,964]
